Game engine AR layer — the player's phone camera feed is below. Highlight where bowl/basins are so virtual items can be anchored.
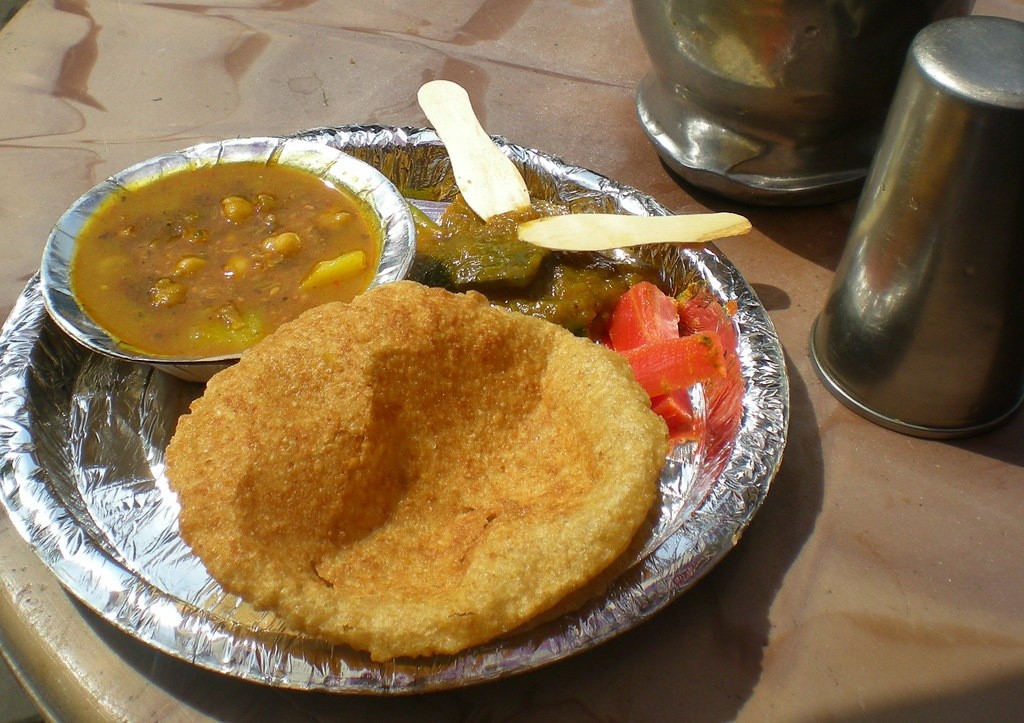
[40,137,418,382]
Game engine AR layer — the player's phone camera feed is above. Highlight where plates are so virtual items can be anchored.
[0,125,791,693]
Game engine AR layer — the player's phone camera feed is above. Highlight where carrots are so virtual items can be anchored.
[600,283,726,429]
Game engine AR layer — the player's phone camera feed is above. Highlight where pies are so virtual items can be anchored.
[163,281,667,661]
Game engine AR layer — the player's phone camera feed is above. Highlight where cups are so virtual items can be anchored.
[808,15,1024,440]
